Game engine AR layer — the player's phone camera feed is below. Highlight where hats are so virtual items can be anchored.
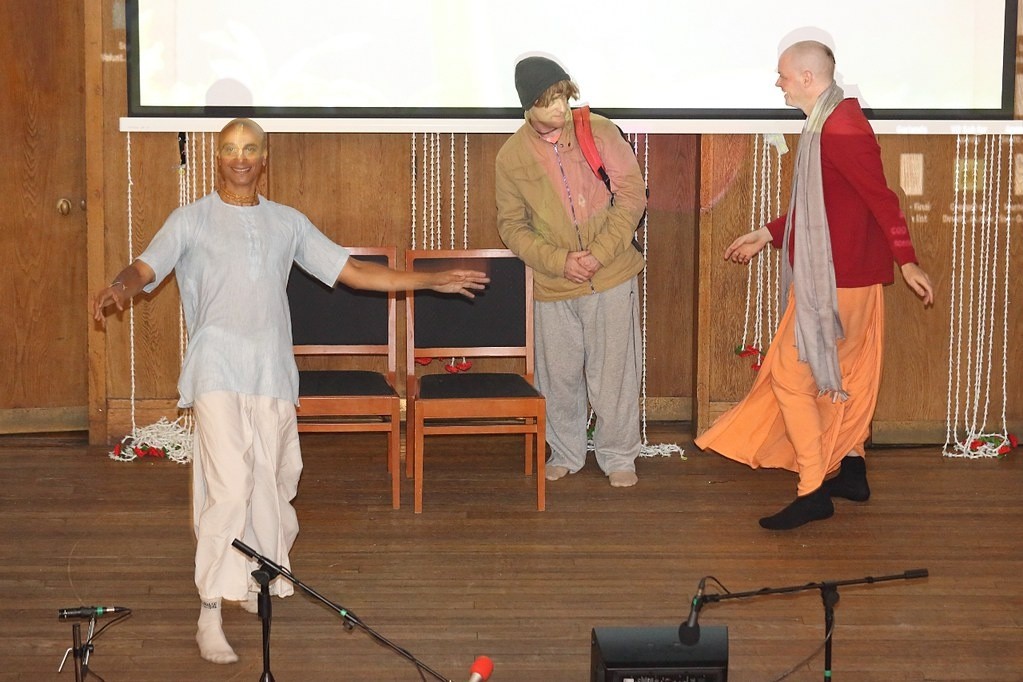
[514,57,570,109]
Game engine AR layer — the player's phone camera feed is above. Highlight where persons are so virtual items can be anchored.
[721,38,936,531]
[92,115,491,662]
[493,55,649,488]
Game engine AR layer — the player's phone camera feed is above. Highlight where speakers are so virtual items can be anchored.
[590,627,729,682]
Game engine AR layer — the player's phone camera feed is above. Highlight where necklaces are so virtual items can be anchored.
[220,185,260,205]
[529,118,559,137]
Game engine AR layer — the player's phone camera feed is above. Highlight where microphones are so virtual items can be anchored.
[679,578,706,646]
[467,656,493,682]
[58,607,129,619]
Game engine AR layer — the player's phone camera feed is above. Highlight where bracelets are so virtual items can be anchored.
[109,281,131,295]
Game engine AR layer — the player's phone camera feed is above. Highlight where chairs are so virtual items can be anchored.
[284,245,399,507]
[407,246,548,512]
